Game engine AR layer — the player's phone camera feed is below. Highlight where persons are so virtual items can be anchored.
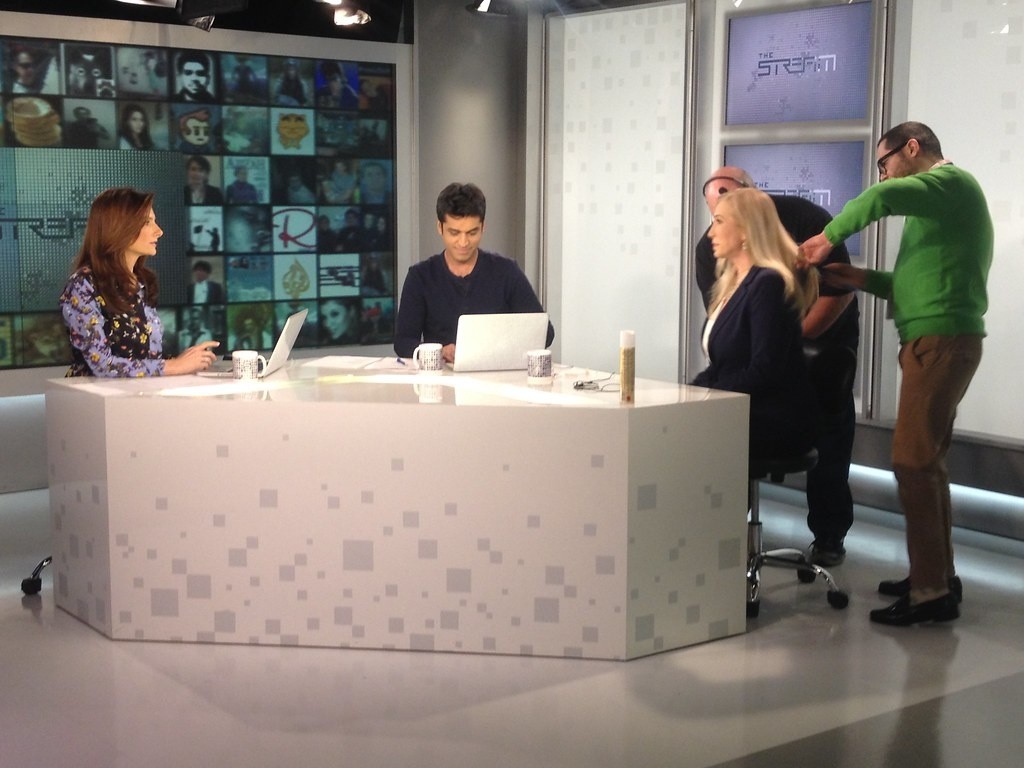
[118,102,162,151]
[187,259,223,304]
[60,186,220,379]
[318,61,393,112]
[322,160,389,206]
[8,45,43,93]
[688,189,821,476]
[319,301,358,344]
[176,49,213,102]
[360,254,389,298]
[358,301,393,344]
[177,309,212,355]
[225,165,259,207]
[182,153,222,205]
[693,166,860,568]
[321,210,389,254]
[795,120,995,629]
[213,55,261,155]
[284,171,314,204]
[274,56,312,108]
[66,101,110,146]
[394,182,555,358]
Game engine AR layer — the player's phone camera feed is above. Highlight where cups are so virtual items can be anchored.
[413,383,444,404]
[527,349,552,386]
[413,343,444,373]
[232,350,266,380]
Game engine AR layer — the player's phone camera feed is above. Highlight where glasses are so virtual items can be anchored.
[16,61,39,70]
[877,137,920,174]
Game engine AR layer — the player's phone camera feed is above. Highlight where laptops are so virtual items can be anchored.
[198,308,308,377]
[446,313,550,372]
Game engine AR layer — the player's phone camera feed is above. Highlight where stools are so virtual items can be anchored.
[747,445,847,617]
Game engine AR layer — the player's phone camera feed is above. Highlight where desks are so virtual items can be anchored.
[45,358,749,660]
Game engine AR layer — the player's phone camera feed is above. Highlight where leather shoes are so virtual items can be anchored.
[808,537,846,566]
[869,591,960,627]
[879,576,963,602]
